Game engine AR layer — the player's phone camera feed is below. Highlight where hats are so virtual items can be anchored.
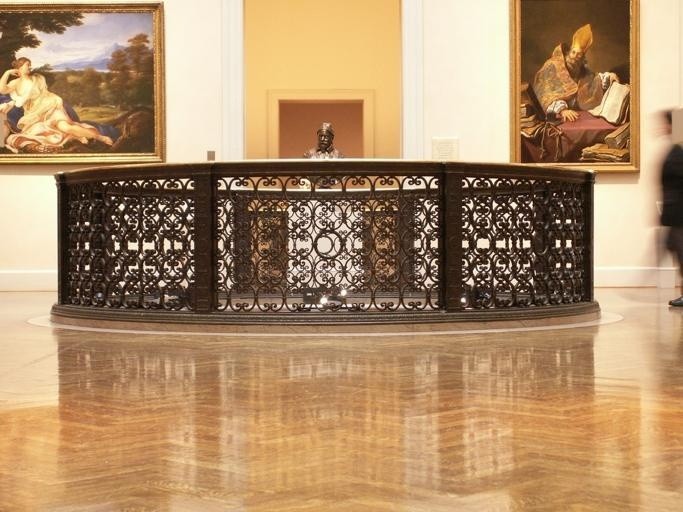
[571,23,592,53]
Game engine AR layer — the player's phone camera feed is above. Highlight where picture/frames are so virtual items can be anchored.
[0,2,166,166]
[510,0,642,174]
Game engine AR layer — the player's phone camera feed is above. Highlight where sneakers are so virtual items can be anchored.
[668,296,683,306]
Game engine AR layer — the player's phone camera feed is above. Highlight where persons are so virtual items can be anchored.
[0,57,114,149]
[533,21,614,122]
[648,106,682,307]
[303,120,347,158]
